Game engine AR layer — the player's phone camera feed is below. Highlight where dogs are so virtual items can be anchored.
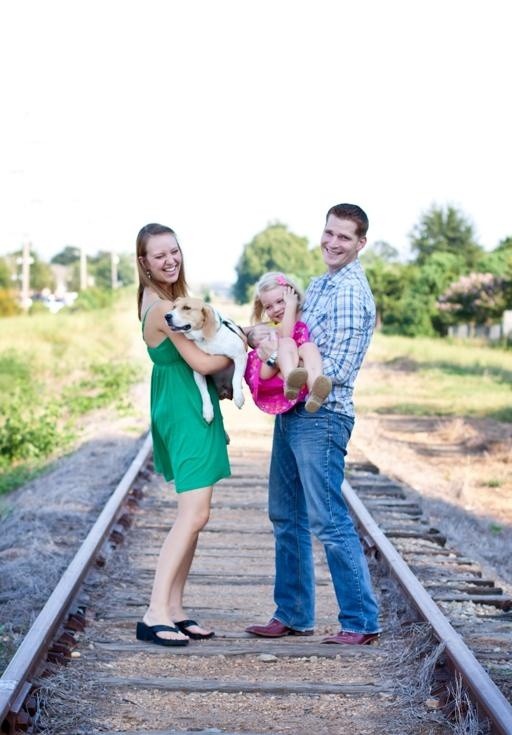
[164,295,248,424]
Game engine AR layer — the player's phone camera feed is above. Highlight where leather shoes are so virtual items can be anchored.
[245,618,314,637]
[321,631,379,646]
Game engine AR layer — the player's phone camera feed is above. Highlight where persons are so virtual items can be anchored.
[246,203,376,645]
[135,223,247,646]
[247,274,333,413]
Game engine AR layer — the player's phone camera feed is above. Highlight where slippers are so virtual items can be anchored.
[175,620,215,640]
[137,621,188,646]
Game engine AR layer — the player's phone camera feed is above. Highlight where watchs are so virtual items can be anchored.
[266,353,278,370]
